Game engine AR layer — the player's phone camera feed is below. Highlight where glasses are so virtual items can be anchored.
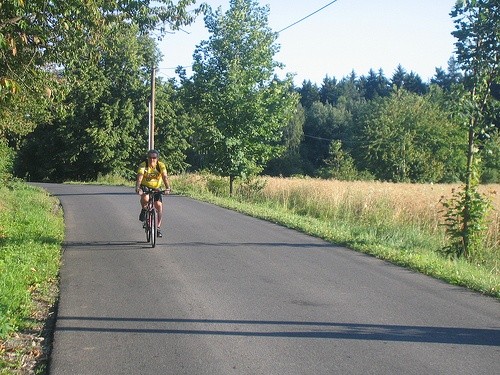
[149,156,157,159]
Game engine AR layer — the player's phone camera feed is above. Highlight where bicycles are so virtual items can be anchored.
[138,188,171,247]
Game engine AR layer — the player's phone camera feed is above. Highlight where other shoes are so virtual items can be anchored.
[158,227,163,238]
[139,207,148,222]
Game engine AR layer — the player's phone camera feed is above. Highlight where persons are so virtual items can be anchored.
[135,149,171,239]
